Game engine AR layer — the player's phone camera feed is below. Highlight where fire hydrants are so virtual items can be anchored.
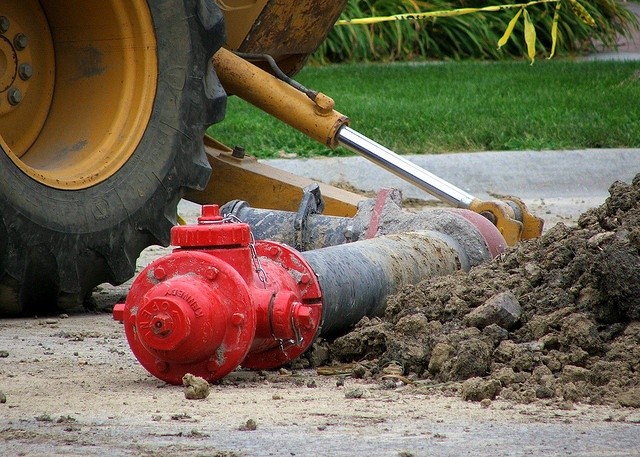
[112,181,507,387]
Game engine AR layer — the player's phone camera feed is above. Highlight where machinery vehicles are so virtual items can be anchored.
[1,0,545,384]
